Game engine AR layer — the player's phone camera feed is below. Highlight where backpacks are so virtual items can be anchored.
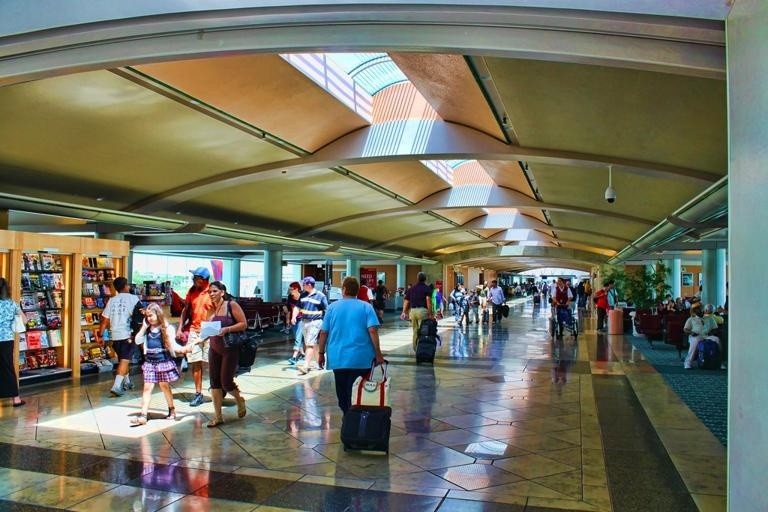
[131,300,144,334]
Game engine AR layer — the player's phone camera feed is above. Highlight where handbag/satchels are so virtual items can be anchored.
[181,303,193,330]
[340,357,394,456]
[237,334,259,368]
[349,361,393,408]
[502,303,510,319]
[418,319,438,336]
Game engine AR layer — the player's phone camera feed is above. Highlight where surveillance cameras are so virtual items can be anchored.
[605,187,616,203]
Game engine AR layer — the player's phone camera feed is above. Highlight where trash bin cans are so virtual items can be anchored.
[607,309,624,335]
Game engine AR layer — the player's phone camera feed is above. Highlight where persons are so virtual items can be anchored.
[400,271,620,352]
[175,265,212,407]
[130,303,186,426]
[371,279,386,324]
[357,278,373,302]
[655,282,728,370]
[291,276,328,374]
[318,276,384,415]
[187,280,248,427]
[0,276,28,408]
[98,276,144,398]
[286,281,304,365]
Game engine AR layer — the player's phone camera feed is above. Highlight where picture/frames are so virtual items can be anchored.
[681,273,693,287]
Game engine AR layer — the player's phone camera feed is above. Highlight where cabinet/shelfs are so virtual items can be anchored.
[0,229,130,387]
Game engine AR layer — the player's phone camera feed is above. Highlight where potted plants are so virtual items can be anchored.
[622,258,673,334]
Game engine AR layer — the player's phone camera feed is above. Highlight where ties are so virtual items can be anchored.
[700,319,705,326]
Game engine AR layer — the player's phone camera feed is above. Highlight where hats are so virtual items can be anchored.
[303,277,316,284]
[189,265,210,280]
[286,281,300,295]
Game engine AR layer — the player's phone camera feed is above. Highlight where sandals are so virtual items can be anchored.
[237,396,247,418]
[127,414,148,426]
[206,415,225,426]
[165,405,177,420]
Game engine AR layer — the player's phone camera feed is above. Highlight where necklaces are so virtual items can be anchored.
[216,301,224,318]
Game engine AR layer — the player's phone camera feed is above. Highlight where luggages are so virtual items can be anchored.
[697,339,720,371]
[415,336,437,365]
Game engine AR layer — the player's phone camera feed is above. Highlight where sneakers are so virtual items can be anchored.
[123,379,137,390]
[109,384,125,397]
[189,392,205,406]
[287,354,312,374]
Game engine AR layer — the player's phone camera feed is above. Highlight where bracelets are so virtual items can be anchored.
[227,326,230,333]
[318,351,325,354]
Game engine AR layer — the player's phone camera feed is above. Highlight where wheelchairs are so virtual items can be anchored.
[548,304,579,341]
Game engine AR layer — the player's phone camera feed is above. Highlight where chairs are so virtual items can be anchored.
[623,296,728,359]
[234,297,288,332]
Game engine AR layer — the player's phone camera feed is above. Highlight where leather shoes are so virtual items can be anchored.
[683,364,693,370]
[12,400,25,407]
[720,363,727,370]
